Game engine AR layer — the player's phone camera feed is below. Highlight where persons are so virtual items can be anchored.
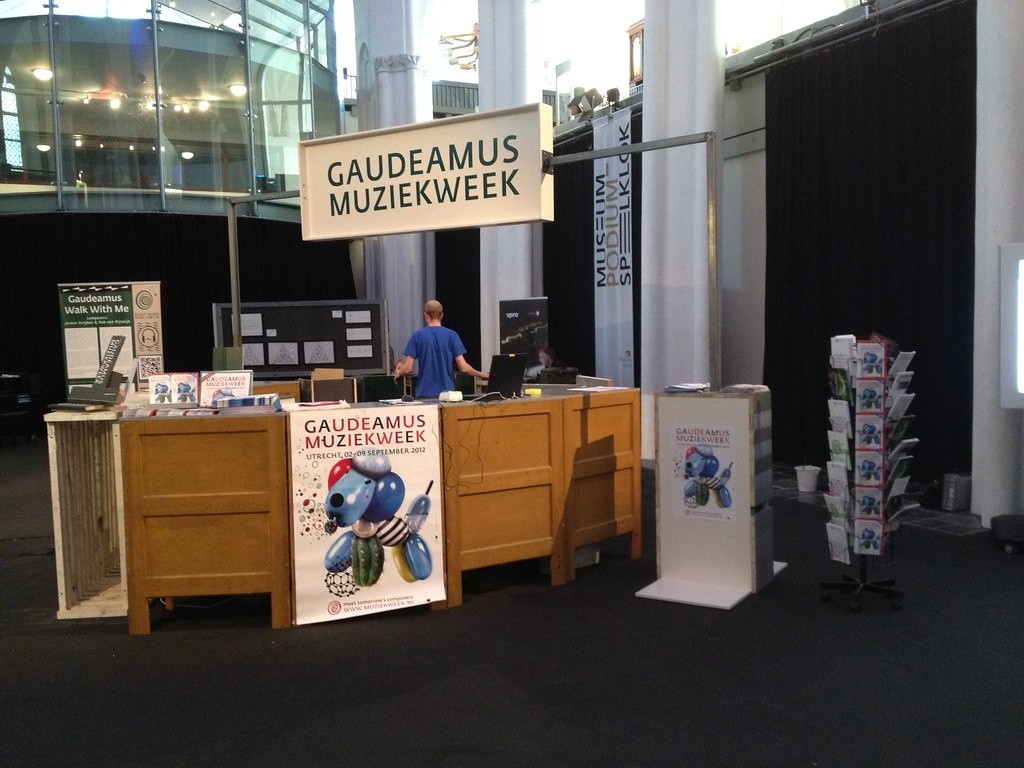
[536,344,573,383]
[395,301,494,401]
[394,357,409,376]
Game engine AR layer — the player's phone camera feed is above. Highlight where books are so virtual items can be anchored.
[46,367,128,414]
[822,331,922,566]
[214,393,282,416]
[663,381,712,394]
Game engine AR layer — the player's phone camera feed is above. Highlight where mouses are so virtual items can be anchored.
[402,395,414,402]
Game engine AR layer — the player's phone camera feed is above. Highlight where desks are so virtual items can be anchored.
[445,384,640,606]
[120,413,291,634]
[636,389,787,610]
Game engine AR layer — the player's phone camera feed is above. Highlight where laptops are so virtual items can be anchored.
[463,352,529,402]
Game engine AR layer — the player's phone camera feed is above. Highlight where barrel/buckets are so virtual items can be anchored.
[794,455,822,493]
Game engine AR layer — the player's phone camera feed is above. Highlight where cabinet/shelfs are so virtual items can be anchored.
[819,331,919,609]
